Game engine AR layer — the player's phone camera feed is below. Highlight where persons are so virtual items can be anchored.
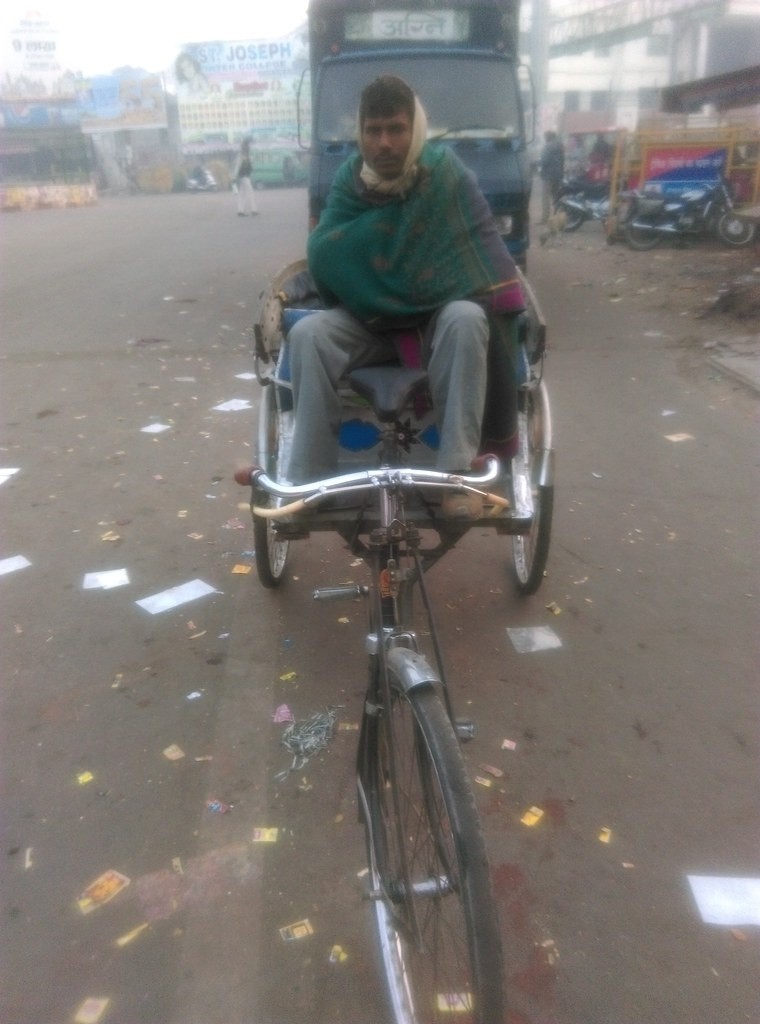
[271,75,521,538]
[232,135,263,217]
[590,132,614,161]
[532,131,565,224]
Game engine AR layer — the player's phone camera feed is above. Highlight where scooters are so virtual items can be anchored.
[186,167,219,192]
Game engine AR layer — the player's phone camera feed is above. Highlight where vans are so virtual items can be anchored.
[249,149,302,190]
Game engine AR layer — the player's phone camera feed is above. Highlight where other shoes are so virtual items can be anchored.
[441,487,486,522]
[275,484,318,526]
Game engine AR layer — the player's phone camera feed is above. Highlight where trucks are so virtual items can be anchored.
[308,0,534,279]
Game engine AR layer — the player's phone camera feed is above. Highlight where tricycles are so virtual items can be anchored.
[234,260,555,1024]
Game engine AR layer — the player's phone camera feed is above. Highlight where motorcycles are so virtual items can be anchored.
[619,173,756,251]
[552,177,634,241]
